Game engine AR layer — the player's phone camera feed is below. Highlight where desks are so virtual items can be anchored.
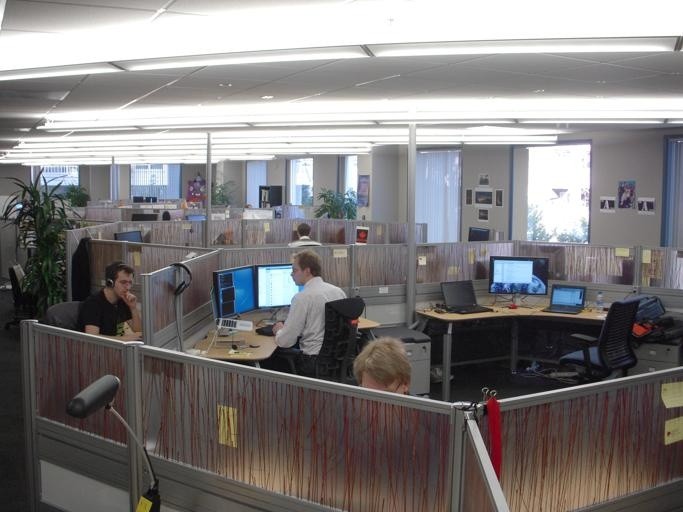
[182,304,378,376]
[415,298,635,402]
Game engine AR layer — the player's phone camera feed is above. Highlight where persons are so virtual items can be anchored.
[272,250,348,375]
[354,337,410,395]
[287,223,322,248]
[77,262,143,341]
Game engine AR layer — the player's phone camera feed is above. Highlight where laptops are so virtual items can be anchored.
[210,288,253,332]
[541,284,585,315]
[441,280,494,314]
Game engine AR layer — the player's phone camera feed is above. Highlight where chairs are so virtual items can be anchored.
[276,292,365,380]
[4,263,33,332]
[43,297,85,330]
[553,298,641,383]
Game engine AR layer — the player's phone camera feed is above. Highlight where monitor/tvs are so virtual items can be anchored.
[213,264,255,319]
[133,196,143,202]
[133,214,156,221]
[146,196,157,203]
[14,202,23,217]
[488,256,549,295]
[257,264,304,311]
[114,231,143,243]
[186,216,206,221]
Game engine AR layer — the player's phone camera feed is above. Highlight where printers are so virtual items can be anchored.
[373,327,430,395]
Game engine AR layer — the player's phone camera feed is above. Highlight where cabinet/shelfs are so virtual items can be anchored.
[258,184,282,209]
[630,329,682,382]
[369,323,432,396]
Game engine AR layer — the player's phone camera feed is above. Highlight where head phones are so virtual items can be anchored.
[169,263,193,295]
[105,277,114,287]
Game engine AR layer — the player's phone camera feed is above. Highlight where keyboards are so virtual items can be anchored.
[256,322,283,336]
